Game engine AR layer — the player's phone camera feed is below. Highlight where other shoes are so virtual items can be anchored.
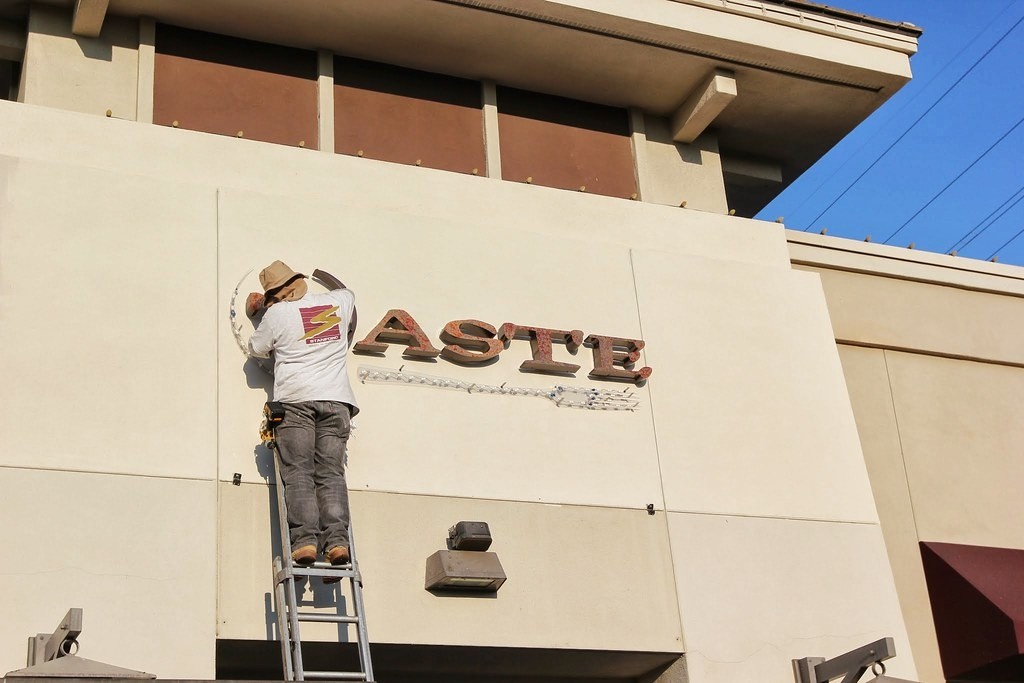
[323,546,349,585]
[291,545,317,582]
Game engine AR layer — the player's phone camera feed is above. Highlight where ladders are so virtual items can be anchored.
[262,397,372,682]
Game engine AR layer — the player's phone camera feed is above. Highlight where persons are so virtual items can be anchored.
[247,261,360,584]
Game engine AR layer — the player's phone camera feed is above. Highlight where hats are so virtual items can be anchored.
[258,260,308,308]
